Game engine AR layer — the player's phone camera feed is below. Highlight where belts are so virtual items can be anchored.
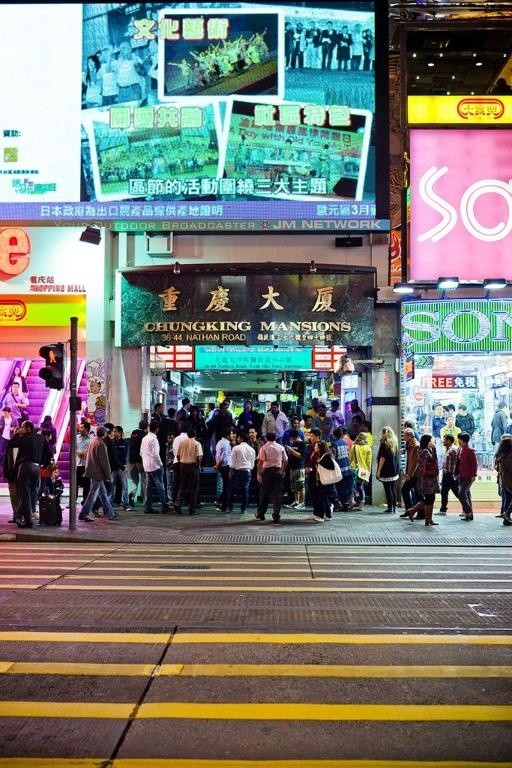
[28,461,39,466]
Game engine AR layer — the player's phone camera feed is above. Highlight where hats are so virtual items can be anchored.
[97,427,110,432]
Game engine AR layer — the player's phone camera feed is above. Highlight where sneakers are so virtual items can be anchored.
[79,501,332,522]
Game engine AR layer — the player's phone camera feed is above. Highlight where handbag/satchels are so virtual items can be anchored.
[422,462,438,477]
[354,467,370,484]
[21,411,28,421]
[317,457,343,485]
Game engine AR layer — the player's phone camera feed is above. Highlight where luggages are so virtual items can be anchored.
[39,494,62,526]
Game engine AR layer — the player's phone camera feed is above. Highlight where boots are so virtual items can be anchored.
[405,502,424,522]
[424,505,438,525]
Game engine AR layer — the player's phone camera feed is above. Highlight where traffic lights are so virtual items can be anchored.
[40,342,63,389]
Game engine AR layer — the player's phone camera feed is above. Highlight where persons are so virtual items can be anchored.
[75,397,512,526]
[82,37,159,110]
[1,367,69,528]
[166,26,272,90]
[284,21,376,74]
